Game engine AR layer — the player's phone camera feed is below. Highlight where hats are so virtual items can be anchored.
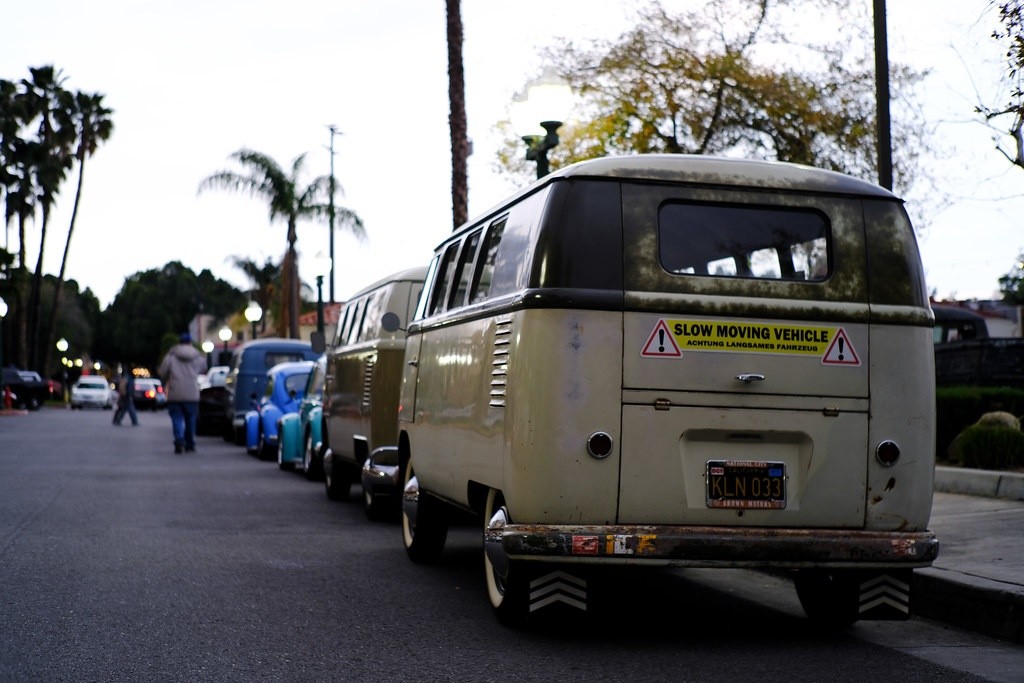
[179,333,191,343]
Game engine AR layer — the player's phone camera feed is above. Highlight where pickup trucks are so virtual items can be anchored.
[931,305,1024,387]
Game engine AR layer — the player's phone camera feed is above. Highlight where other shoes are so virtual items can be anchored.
[174,441,195,454]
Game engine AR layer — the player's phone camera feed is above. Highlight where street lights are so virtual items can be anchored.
[243,302,263,339]
[218,327,232,365]
[56,337,69,403]
[201,340,214,371]
[309,254,332,345]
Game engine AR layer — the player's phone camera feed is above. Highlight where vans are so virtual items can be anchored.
[396,152,941,629]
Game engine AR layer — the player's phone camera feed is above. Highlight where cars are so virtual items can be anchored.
[134,377,167,412]
[0,365,48,411]
[69,374,114,411]
[194,265,430,518]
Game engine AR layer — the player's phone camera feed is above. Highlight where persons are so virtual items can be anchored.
[112,366,141,427]
[158,332,212,453]
